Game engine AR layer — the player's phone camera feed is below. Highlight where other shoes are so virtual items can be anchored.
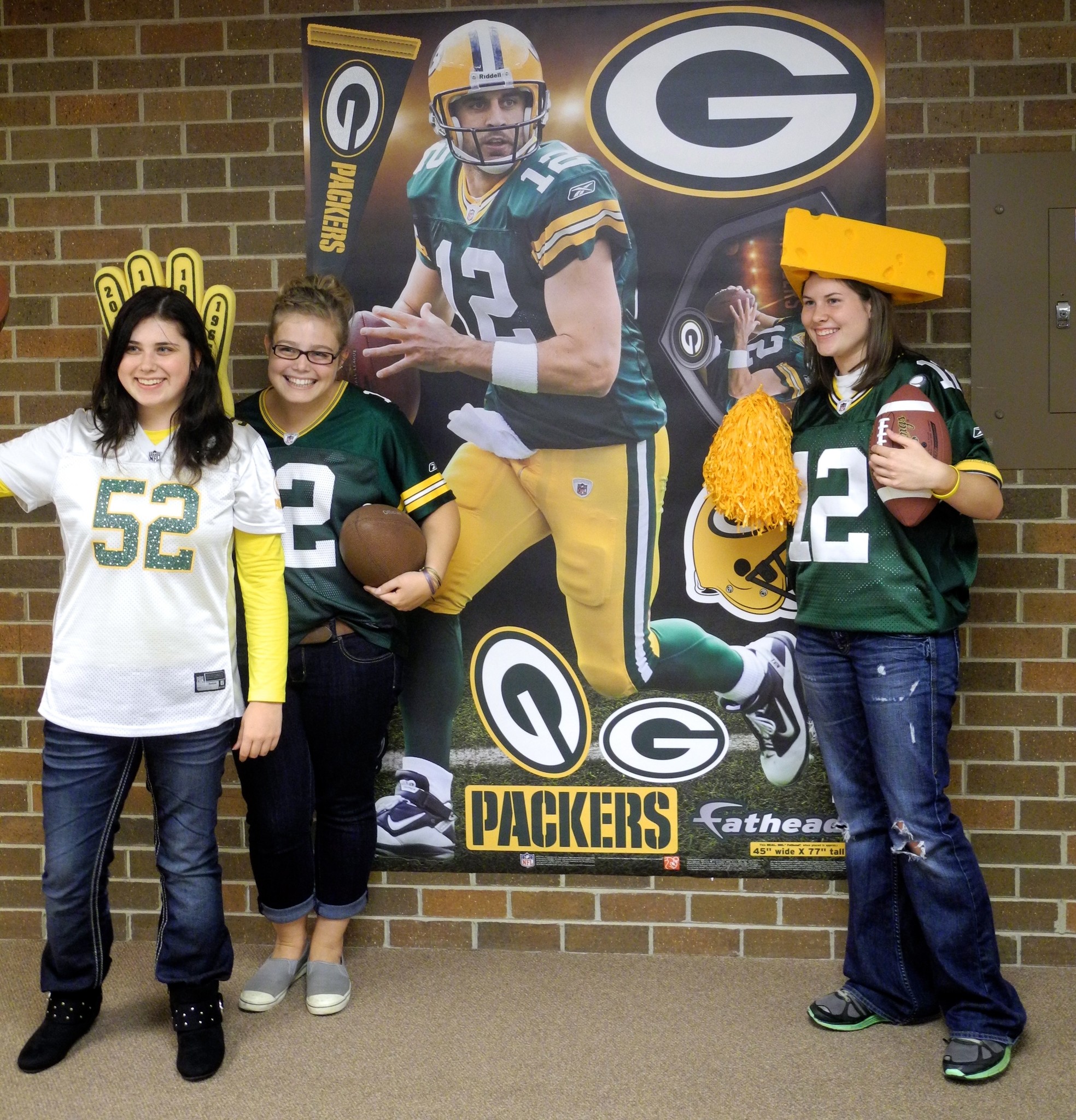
[18,986,102,1074]
[169,975,225,1083]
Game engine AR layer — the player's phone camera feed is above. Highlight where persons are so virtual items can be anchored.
[781,273,1028,1081]
[356,19,810,861]
[714,285,818,424]
[231,274,460,1018]
[0,286,289,1083]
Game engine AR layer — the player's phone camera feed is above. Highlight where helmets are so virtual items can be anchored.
[428,19,550,175]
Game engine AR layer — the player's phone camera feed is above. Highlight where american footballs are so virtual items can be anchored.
[338,502,426,588]
[703,288,755,323]
[867,384,953,526]
[346,310,422,427]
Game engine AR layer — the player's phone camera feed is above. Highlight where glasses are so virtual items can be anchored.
[271,341,346,366]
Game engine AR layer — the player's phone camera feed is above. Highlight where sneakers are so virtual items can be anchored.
[806,991,893,1030]
[306,948,351,1014]
[374,767,458,862]
[942,1034,1012,1081]
[717,630,814,787]
[239,939,309,1012]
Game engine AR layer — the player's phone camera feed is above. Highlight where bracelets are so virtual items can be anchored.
[931,465,960,499]
[420,566,441,594]
[492,341,538,394]
[728,350,748,369]
